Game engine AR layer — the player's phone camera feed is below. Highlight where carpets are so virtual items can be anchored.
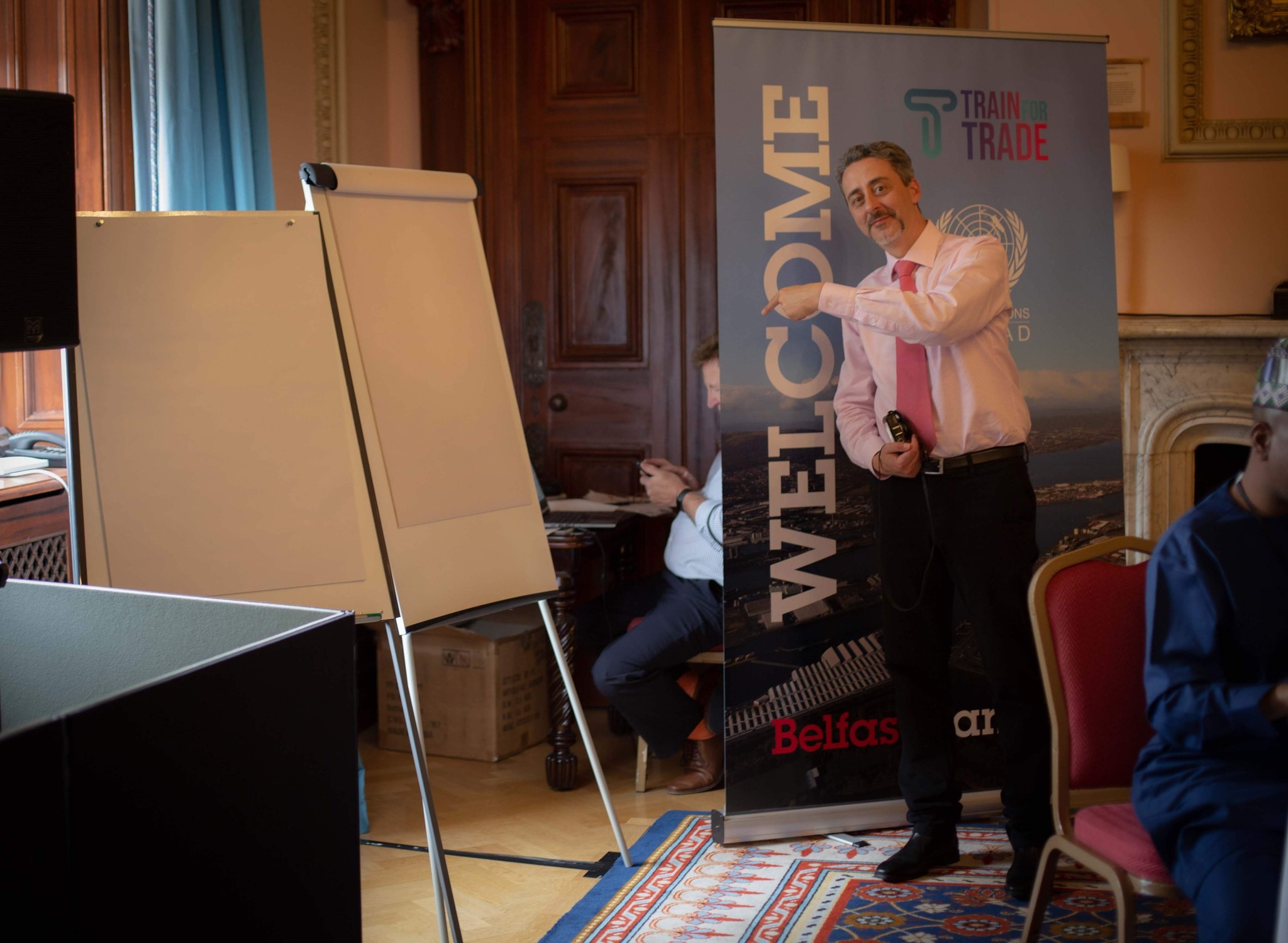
[540,809,1199,943]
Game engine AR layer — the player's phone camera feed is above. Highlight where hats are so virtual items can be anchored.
[1253,338,1288,411]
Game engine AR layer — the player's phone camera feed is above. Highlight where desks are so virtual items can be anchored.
[544,514,644,795]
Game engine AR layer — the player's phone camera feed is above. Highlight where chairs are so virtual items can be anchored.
[1019,536,1176,943]
[628,616,724,797]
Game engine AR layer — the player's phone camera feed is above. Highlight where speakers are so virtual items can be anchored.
[0,86,80,353]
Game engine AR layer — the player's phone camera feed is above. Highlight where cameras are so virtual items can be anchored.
[883,412,912,456]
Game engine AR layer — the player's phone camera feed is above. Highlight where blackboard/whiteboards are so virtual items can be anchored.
[299,162,559,635]
[61,209,403,623]
[5,430,68,468]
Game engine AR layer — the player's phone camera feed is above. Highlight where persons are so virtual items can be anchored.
[591,331,724,794]
[1131,337,1288,943]
[760,141,1062,907]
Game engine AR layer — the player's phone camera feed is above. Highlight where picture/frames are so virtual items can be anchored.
[1160,0,1288,162]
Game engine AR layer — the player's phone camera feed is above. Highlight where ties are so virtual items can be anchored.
[894,261,938,457]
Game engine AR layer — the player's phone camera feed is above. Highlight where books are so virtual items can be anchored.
[543,511,625,535]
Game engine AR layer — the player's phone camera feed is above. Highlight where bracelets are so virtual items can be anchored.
[676,488,694,511]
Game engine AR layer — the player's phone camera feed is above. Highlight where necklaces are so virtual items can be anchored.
[1236,471,1288,513]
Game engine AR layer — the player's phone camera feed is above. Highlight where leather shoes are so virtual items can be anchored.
[1003,850,1043,899]
[645,732,723,795]
[874,821,960,882]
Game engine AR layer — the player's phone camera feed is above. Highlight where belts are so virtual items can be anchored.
[919,445,1025,474]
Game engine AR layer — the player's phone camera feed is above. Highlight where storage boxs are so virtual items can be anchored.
[372,604,546,767]
